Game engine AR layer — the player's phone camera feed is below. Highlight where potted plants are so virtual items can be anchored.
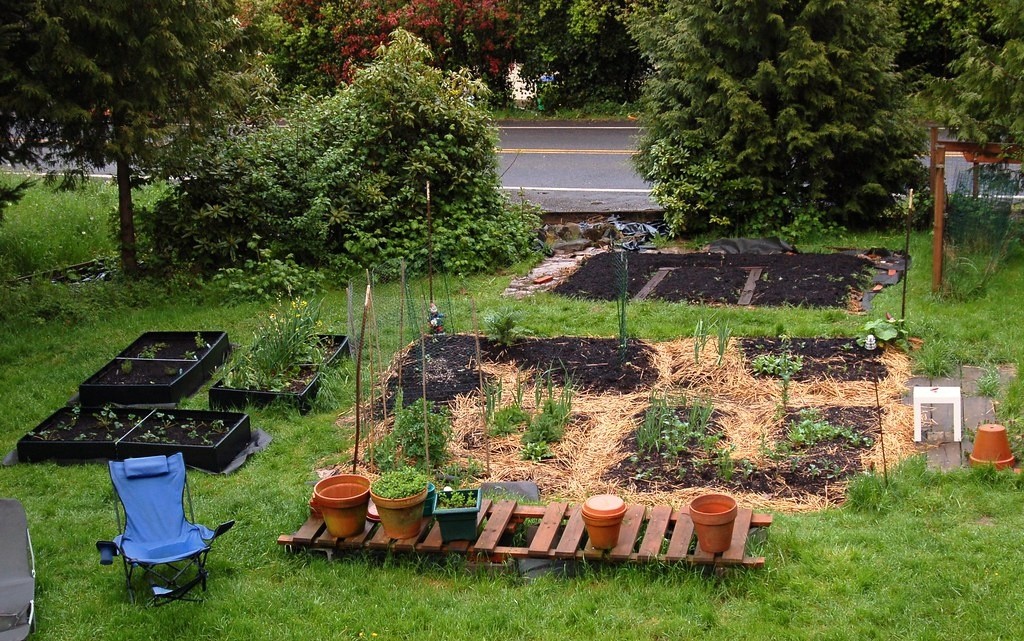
[432,488,482,542]
[370,467,428,540]
[16,291,351,473]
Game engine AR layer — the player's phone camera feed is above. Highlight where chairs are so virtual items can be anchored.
[0,498,36,641]
[96,452,236,610]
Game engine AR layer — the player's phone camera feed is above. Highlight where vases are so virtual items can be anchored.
[581,494,628,550]
[689,494,737,553]
[969,424,1015,471]
[314,473,370,538]
